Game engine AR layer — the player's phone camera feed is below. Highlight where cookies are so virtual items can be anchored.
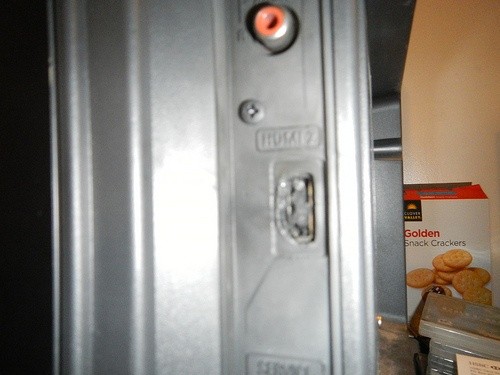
[406,249,492,306]
